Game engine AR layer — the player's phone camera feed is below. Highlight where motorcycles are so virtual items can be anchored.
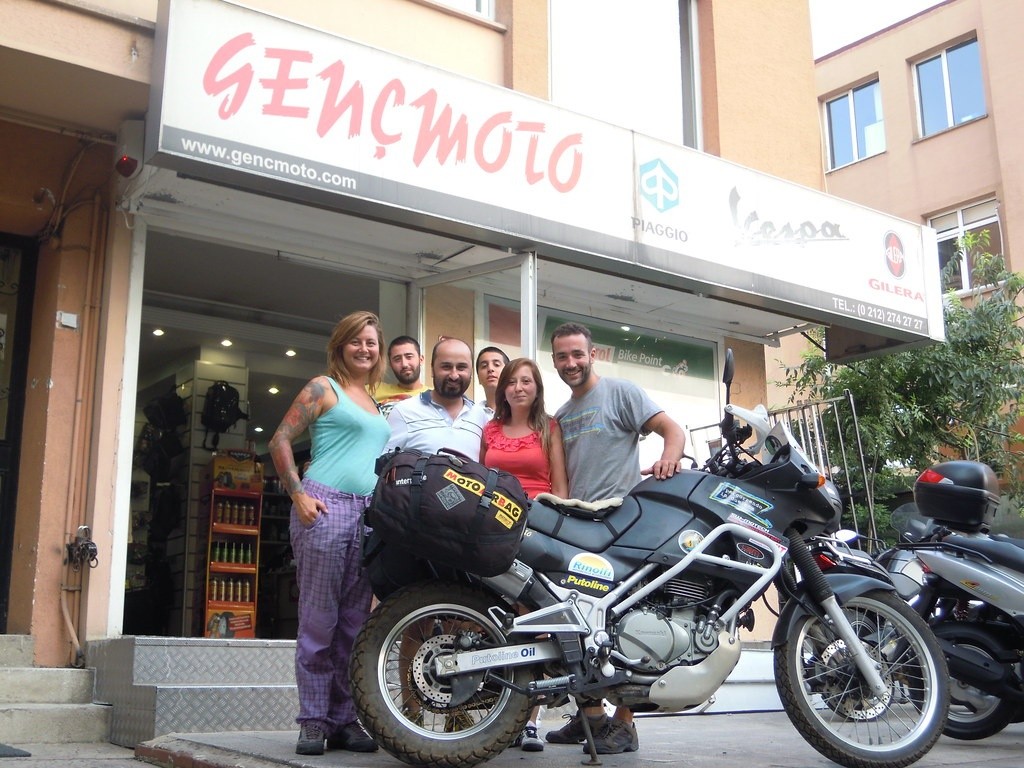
[346,348,953,768]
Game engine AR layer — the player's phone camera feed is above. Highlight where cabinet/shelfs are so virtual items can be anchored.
[205,450,264,639]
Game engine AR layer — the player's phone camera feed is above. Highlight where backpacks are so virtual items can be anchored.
[135,386,187,635]
[364,447,531,578]
[201,380,242,452]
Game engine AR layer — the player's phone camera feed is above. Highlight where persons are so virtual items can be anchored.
[269,311,392,755]
[365,336,434,420]
[476,347,510,419]
[478,357,567,751]
[546,322,685,754]
[382,339,489,732]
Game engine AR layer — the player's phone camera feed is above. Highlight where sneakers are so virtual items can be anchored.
[444,713,474,732]
[520,726,544,751]
[295,724,325,755]
[404,711,425,729]
[508,735,521,748]
[546,710,609,744]
[327,720,379,751]
[583,717,639,754]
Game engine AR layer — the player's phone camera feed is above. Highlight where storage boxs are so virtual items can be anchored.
[913,461,1001,528]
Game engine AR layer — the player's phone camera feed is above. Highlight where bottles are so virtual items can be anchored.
[212,541,252,565]
[215,500,256,526]
[208,577,250,603]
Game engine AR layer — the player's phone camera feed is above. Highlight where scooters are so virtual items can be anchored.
[785,459,1024,741]
